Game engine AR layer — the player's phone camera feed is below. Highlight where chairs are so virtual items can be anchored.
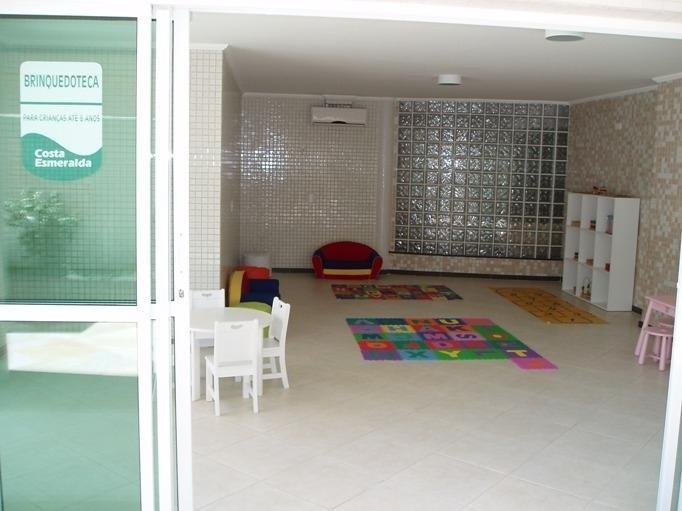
[190,288,291,416]
[228,270,279,338]
[312,242,383,280]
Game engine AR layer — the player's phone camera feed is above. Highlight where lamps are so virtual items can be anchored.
[438,74,462,86]
[545,29,584,41]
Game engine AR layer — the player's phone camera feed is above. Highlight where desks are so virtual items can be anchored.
[634,296,676,356]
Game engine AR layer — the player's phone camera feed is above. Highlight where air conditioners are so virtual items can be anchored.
[310,106,367,127]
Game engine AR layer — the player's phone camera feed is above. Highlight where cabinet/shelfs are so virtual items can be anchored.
[561,192,640,311]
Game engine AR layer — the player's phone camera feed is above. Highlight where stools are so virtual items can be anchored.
[639,318,674,371]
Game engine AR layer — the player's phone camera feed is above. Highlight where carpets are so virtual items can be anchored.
[492,287,608,325]
[345,317,558,370]
[330,283,463,301]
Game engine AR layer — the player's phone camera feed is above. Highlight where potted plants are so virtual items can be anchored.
[3,185,84,279]
